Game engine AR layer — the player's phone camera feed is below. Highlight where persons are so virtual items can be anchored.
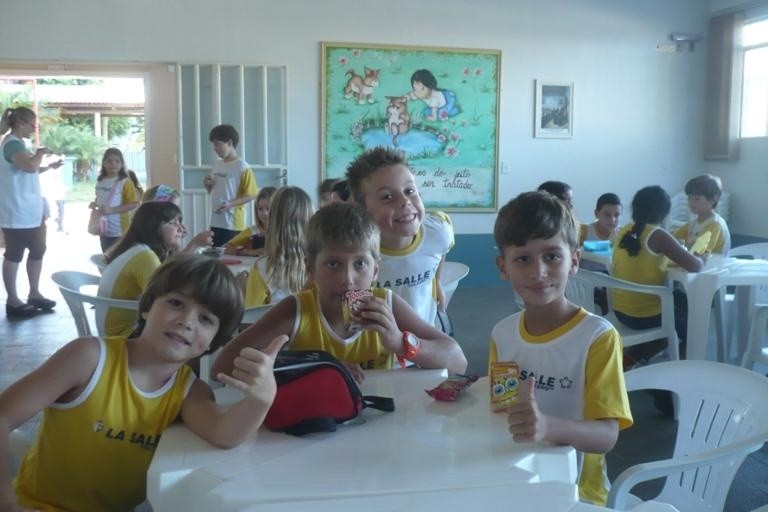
[489,190,635,512]
[329,179,356,206]
[94,202,201,378]
[88,149,137,259]
[669,174,731,260]
[223,187,281,255]
[321,179,342,209]
[612,186,703,371]
[209,204,467,382]
[125,170,144,204]
[537,182,574,216]
[142,185,186,248]
[0,105,65,317]
[0,256,290,512]
[579,193,623,246]
[348,148,456,329]
[246,186,316,308]
[405,70,461,121]
[204,125,256,248]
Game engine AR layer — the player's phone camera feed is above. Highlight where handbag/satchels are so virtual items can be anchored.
[88,180,118,236]
[265,350,395,436]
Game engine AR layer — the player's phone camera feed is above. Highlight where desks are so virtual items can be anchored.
[147,374,580,511]
[667,257,767,359]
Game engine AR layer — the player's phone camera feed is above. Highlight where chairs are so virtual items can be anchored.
[361,368,448,374]
[740,305,768,370]
[200,305,275,415]
[725,242,768,304]
[665,190,730,234]
[51,271,139,336]
[578,360,768,511]
[565,268,680,421]
[91,254,109,276]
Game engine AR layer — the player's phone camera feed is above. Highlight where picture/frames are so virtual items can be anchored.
[321,42,500,212]
[534,79,574,139]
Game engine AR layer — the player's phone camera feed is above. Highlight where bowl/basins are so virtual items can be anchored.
[202,246,225,261]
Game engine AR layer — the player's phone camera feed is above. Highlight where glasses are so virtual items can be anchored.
[22,119,36,129]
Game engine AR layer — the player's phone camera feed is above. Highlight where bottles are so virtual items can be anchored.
[490,361,521,416]
[343,288,374,333]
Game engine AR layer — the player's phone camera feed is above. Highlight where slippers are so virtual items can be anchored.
[6,303,37,317]
[27,299,56,311]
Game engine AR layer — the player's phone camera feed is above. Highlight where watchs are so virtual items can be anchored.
[399,329,417,369]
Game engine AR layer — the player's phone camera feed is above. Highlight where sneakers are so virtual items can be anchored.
[649,389,673,416]
[623,354,637,372]
